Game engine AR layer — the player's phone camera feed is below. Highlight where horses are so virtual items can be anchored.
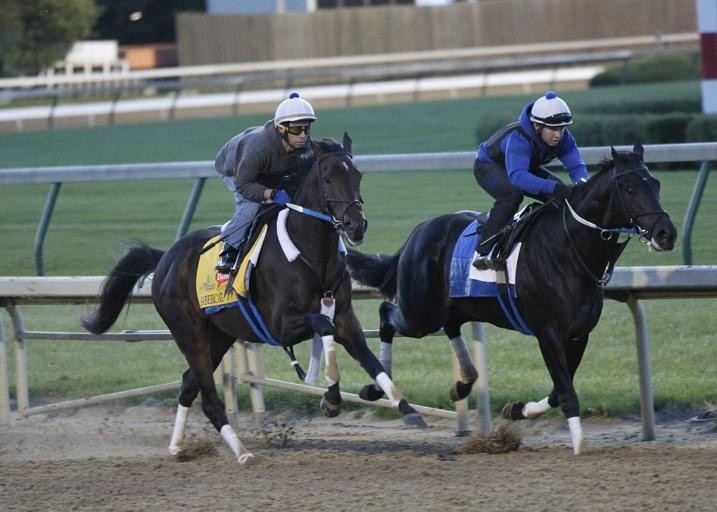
[81,132,428,467]
[346,141,677,456]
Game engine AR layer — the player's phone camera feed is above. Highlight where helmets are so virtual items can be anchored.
[530,92,572,127]
[274,93,317,128]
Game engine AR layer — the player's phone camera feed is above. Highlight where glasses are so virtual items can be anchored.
[278,123,308,135]
[544,113,571,124]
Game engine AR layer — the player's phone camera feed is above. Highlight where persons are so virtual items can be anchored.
[212,89,318,274]
[471,91,591,271]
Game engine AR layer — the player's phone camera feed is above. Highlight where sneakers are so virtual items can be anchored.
[215,253,236,270]
[473,253,504,269]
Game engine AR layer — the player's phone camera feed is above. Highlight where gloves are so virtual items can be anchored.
[271,188,292,207]
[554,182,572,199]
